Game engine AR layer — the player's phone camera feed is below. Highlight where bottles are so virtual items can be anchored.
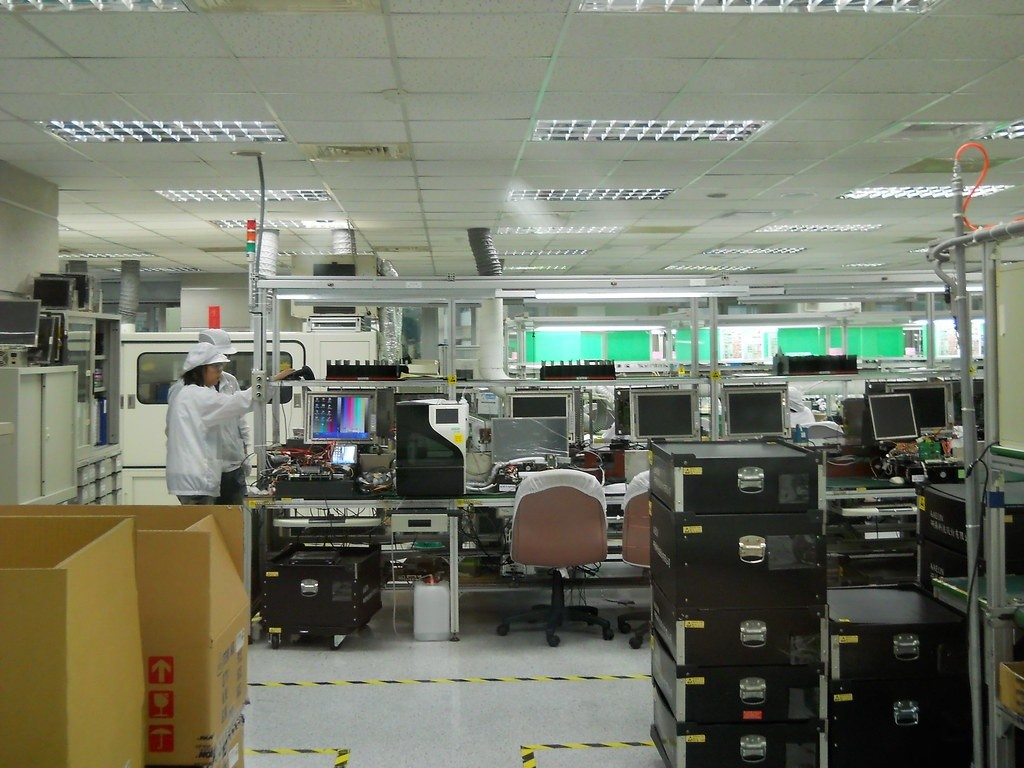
[412,577,450,641]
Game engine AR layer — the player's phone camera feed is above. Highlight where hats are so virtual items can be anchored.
[183,341,230,374]
[199,329,237,354]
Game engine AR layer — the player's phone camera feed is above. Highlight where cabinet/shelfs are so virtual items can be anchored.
[42,310,123,505]
[984,239,1024,768]
[255,276,984,641]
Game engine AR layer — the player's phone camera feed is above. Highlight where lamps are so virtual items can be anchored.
[536,285,750,300]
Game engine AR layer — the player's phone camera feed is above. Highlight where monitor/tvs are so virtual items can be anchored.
[637,395,693,438]
[728,392,782,434]
[893,387,946,427]
[304,391,377,444]
[951,381,985,425]
[870,396,916,437]
[511,396,567,417]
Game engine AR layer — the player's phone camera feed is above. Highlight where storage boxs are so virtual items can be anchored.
[263,541,382,649]
[0,505,250,768]
[824,584,974,768]
[916,483,1024,593]
[644,435,827,768]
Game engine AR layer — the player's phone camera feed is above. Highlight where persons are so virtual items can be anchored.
[164,329,297,506]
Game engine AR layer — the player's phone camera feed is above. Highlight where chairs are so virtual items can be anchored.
[497,469,614,647]
[618,471,652,650]
